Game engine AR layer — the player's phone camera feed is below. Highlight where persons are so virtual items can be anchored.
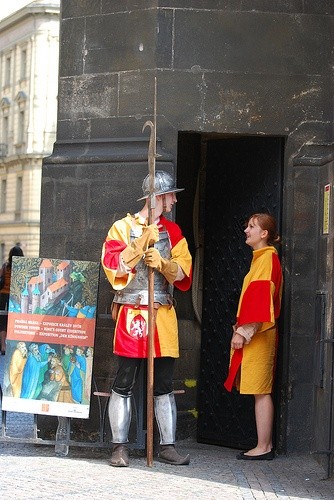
[0,247,24,355]
[224,213,284,461]
[100,171,192,468]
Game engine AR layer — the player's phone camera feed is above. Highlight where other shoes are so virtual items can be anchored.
[158,444,190,465]
[237,446,276,459]
[109,443,130,468]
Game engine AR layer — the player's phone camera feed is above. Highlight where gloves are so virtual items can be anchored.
[121,217,161,269]
[143,248,178,284]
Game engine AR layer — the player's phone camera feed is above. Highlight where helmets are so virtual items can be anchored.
[137,170,184,202]
